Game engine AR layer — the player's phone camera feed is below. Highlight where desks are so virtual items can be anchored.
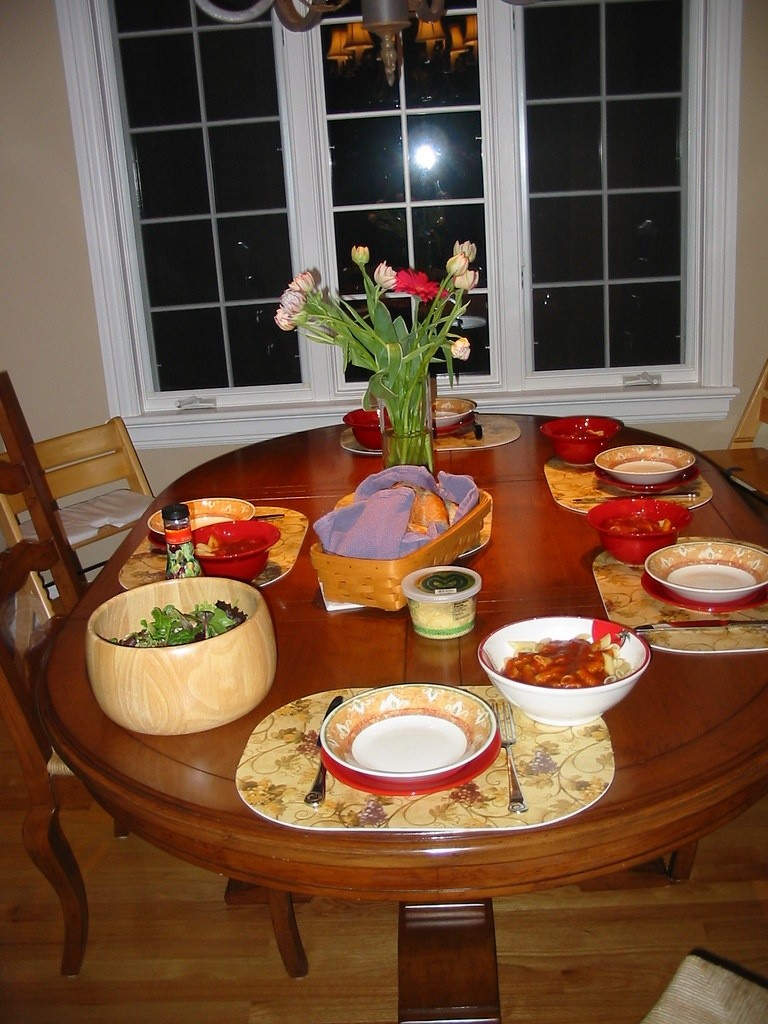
[33,414,768,1023]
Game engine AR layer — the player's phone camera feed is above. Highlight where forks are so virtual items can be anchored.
[491,701,529,814]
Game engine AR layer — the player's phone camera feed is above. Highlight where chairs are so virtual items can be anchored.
[1,369,310,981]
[0,417,156,658]
[666,358,767,884]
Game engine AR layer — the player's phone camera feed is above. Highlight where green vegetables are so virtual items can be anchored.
[109,599,248,647]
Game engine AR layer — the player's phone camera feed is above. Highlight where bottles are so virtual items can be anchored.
[161,504,204,581]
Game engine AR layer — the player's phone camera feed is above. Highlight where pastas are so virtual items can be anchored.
[502,632,635,688]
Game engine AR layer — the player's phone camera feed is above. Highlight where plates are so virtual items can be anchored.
[320,728,501,796]
[148,531,167,552]
[640,573,768,612]
[594,467,700,495]
[434,417,476,437]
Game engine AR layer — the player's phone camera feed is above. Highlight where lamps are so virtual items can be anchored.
[327,0,479,78]
[195,0,543,88]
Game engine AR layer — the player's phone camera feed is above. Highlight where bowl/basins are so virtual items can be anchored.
[586,497,693,566]
[146,497,256,544]
[342,406,393,450]
[85,575,278,737]
[477,615,651,728]
[539,415,624,466]
[402,566,482,639]
[191,519,281,586]
[434,397,478,427]
[644,541,768,603]
[594,443,696,486]
[318,683,497,780]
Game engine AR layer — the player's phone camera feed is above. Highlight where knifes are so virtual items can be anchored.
[473,420,483,441]
[251,514,285,520]
[634,619,768,632]
[303,695,343,808]
[573,491,700,503]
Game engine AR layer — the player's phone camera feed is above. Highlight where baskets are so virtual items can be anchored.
[312,488,491,610]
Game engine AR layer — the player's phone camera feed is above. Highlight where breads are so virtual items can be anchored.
[331,481,460,536]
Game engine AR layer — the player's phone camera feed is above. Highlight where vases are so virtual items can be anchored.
[376,372,434,475]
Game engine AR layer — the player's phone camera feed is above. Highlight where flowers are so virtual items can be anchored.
[274,241,480,472]
